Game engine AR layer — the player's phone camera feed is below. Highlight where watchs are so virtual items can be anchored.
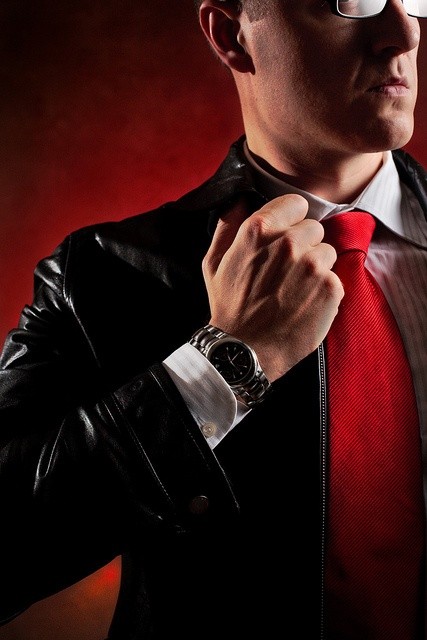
[190,327,272,410]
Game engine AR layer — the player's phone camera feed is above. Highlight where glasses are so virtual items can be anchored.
[335,0,427,18]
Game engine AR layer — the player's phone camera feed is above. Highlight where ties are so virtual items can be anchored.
[319,210,426,640]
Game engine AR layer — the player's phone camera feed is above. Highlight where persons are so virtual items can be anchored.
[0,1,427,638]
